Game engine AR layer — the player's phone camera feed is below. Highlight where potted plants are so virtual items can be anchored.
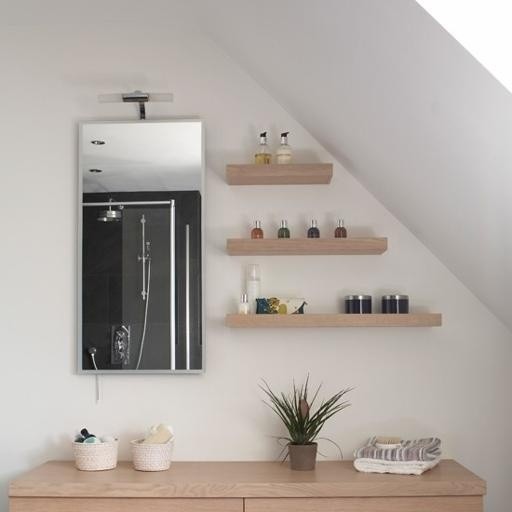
[257,373,358,470]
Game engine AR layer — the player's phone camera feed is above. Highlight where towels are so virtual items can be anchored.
[353,458,441,476]
[353,435,442,462]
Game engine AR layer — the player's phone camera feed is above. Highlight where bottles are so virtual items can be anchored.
[245,263,261,314]
[251,221,264,239]
[307,219,320,238]
[359,295,371,313]
[334,218,347,238]
[344,295,359,313]
[395,294,409,314]
[381,295,395,314]
[277,219,290,240]
[238,293,250,315]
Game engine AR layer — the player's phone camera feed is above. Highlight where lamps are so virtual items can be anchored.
[99,90,173,119]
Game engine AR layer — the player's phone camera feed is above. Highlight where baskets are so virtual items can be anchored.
[73,440,118,470]
[131,436,174,472]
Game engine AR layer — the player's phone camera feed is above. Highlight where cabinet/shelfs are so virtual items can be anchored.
[225,163,442,333]
[7,460,487,512]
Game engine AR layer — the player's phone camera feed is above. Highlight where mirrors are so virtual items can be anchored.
[76,117,206,375]
